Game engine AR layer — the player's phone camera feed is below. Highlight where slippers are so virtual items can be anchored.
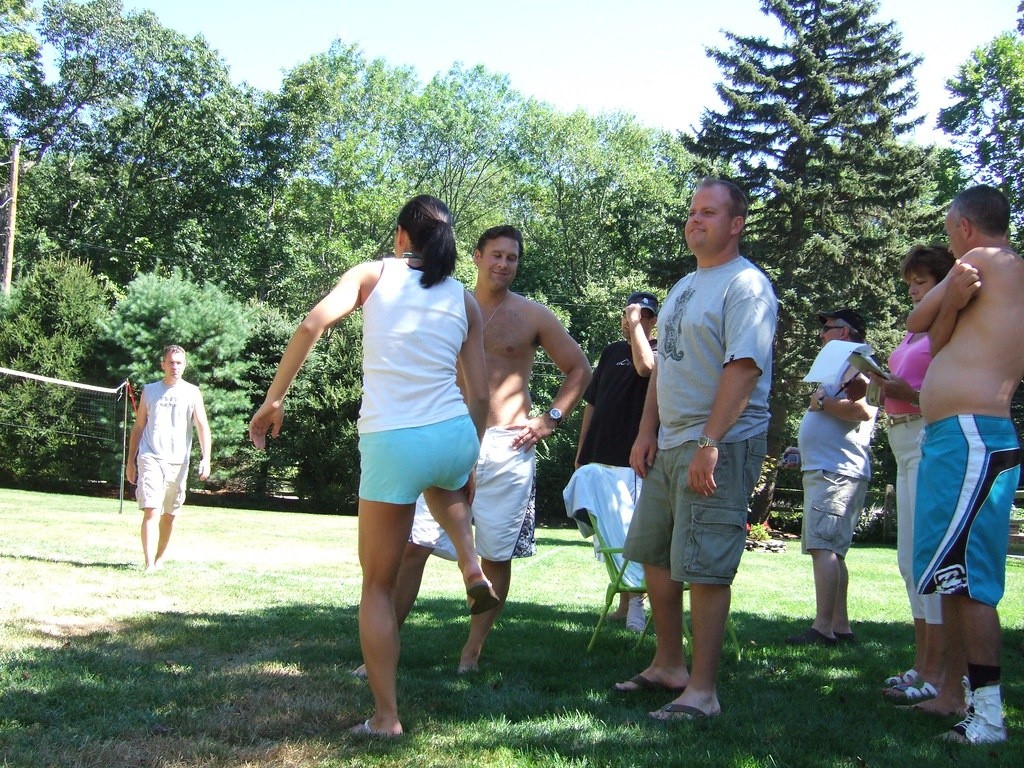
[883,679,938,700]
[833,631,853,642]
[883,668,919,686]
[612,674,686,693]
[785,627,838,646]
[467,581,500,615]
[651,703,708,722]
[346,719,404,740]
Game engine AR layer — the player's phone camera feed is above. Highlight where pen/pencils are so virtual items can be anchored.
[834,370,861,397]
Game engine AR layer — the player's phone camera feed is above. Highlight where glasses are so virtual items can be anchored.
[823,324,844,332]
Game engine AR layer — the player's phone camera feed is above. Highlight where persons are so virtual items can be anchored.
[609,177,778,727]
[250,195,501,743]
[574,292,661,634]
[126,345,211,571]
[867,246,954,703]
[388,225,593,677]
[906,186,1024,747]
[782,308,883,649]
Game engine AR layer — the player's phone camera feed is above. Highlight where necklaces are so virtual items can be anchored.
[400,252,425,260]
[473,290,509,332]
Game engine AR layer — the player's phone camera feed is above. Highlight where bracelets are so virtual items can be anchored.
[909,389,920,408]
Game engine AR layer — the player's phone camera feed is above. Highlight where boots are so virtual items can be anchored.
[932,676,1006,745]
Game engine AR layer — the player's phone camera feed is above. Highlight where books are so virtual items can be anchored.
[802,340,891,386]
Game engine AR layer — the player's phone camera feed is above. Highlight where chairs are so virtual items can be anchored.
[565,461,742,667]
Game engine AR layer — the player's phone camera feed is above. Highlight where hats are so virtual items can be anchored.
[818,309,866,337]
[622,291,659,319]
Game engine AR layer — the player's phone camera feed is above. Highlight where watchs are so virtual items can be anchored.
[815,394,826,410]
[697,433,719,448]
[544,408,564,424]
